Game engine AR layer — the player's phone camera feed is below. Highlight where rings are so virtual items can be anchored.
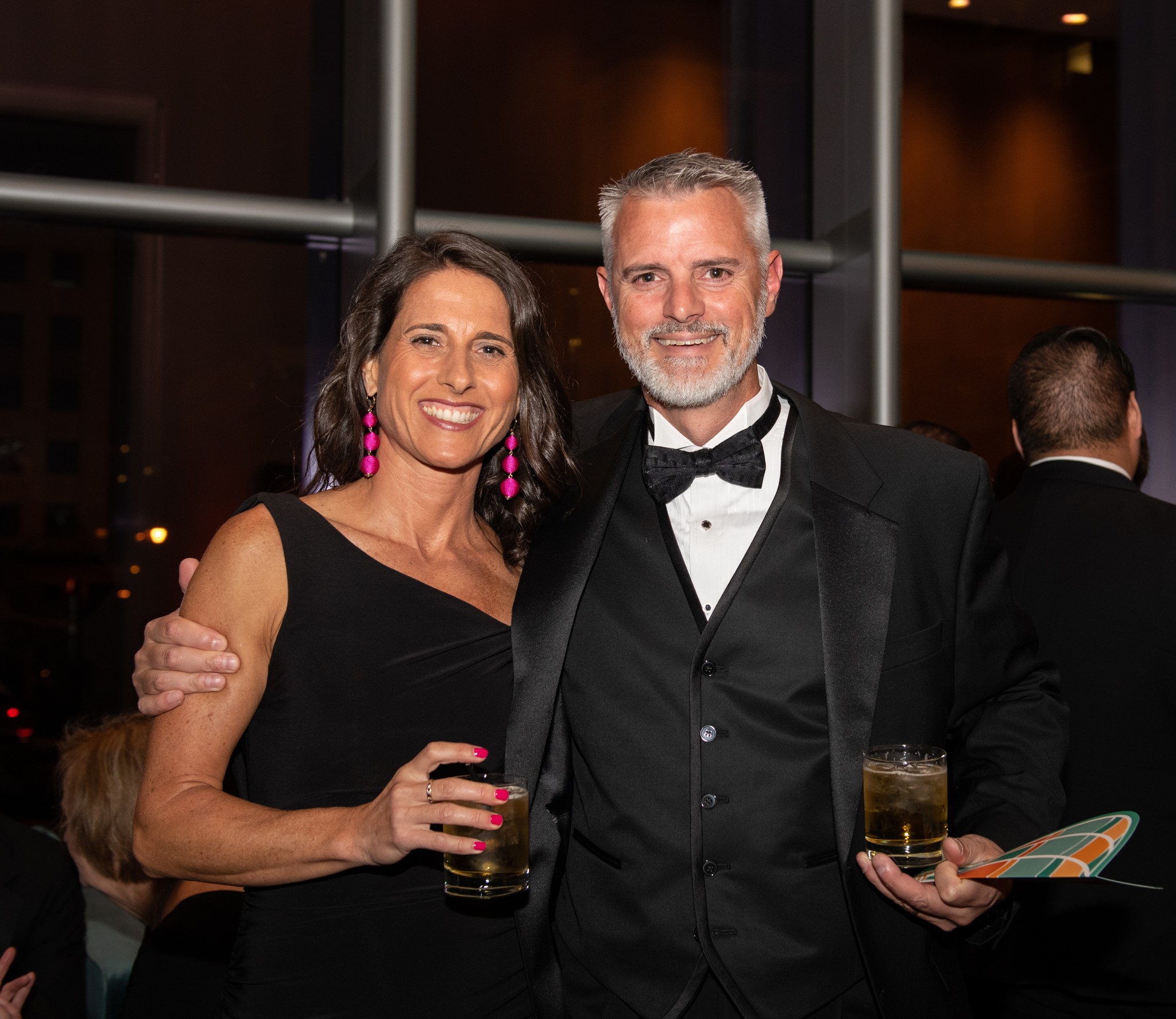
[426,779,436,806]
[912,909,920,917]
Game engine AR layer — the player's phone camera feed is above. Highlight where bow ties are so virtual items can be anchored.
[641,387,782,506]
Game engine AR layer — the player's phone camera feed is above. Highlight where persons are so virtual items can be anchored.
[989,327,1176,1019]
[56,711,182,1016]
[0,946,35,1018]
[129,219,581,1015]
[136,152,1074,1017]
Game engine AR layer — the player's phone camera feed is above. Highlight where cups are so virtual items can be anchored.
[863,743,946,868]
[443,774,530,897]
